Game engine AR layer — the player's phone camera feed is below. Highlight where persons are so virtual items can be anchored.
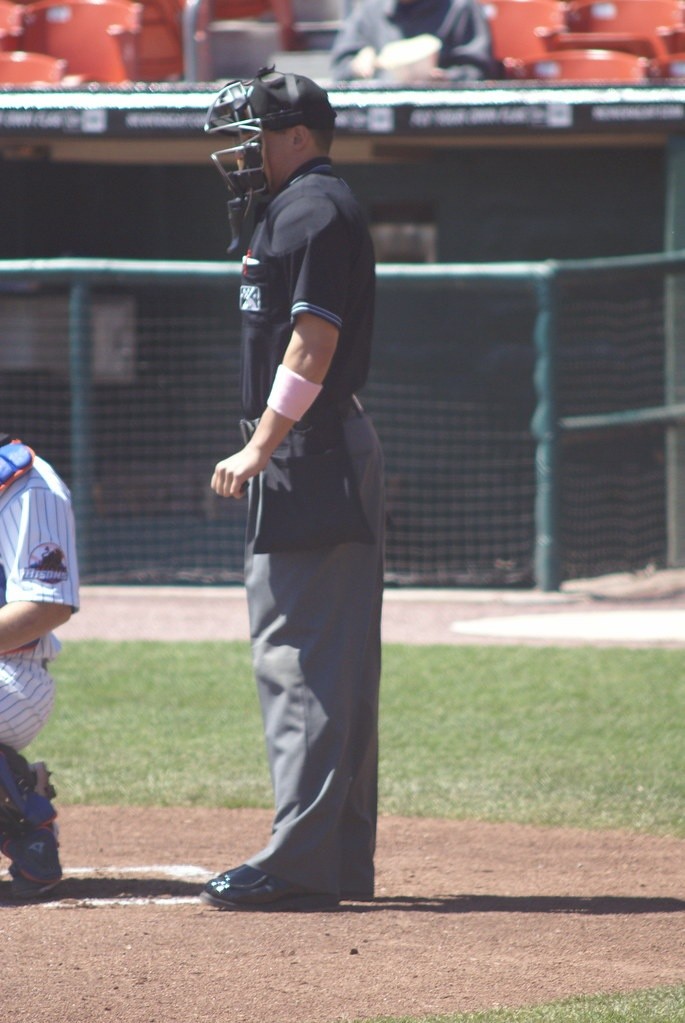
[0,431,80,897]
[198,66,388,912]
[328,0,497,82]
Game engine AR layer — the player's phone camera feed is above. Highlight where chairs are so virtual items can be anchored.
[0,0,685,89]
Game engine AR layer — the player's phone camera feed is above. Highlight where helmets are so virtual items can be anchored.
[209,68,335,136]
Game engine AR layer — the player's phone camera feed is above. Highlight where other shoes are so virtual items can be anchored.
[339,893,373,902]
[3,761,62,899]
[200,864,339,913]
